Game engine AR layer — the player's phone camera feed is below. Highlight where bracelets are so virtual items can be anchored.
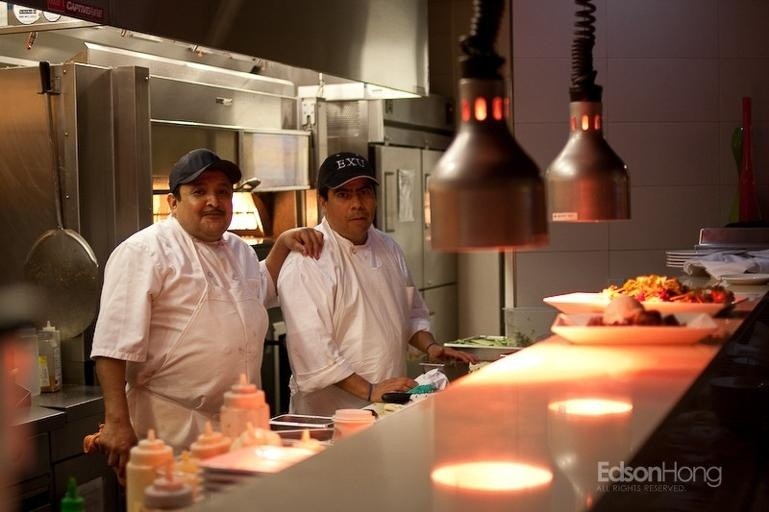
[426,342,437,353]
[367,383,372,401]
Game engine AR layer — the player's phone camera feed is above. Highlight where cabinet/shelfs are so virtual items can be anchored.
[1,430,62,512]
[48,403,123,511]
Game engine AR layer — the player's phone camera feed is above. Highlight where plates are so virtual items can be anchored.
[660,249,712,269]
[717,274,768,286]
[537,291,750,318]
[549,310,718,349]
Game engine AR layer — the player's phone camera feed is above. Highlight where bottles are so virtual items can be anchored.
[227,423,280,452]
[39,320,61,388]
[60,476,85,512]
[124,420,233,511]
[291,430,327,460]
[13,328,41,397]
[219,373,269,439]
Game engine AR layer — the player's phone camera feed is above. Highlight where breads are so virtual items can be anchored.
[603,296,644,326]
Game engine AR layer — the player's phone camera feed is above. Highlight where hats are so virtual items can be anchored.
[318,151,379,192]
[169,149,241,192]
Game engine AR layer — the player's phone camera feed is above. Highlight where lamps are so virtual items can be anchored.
[525,1,635,224]
[412,1,552,260]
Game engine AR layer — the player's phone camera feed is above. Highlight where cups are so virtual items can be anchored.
[330,407,372,441]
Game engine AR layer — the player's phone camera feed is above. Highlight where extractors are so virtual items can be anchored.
[0,1,432,104]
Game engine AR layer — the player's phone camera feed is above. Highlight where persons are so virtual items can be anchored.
[277,152,479,416]
[90,148,323,479]
